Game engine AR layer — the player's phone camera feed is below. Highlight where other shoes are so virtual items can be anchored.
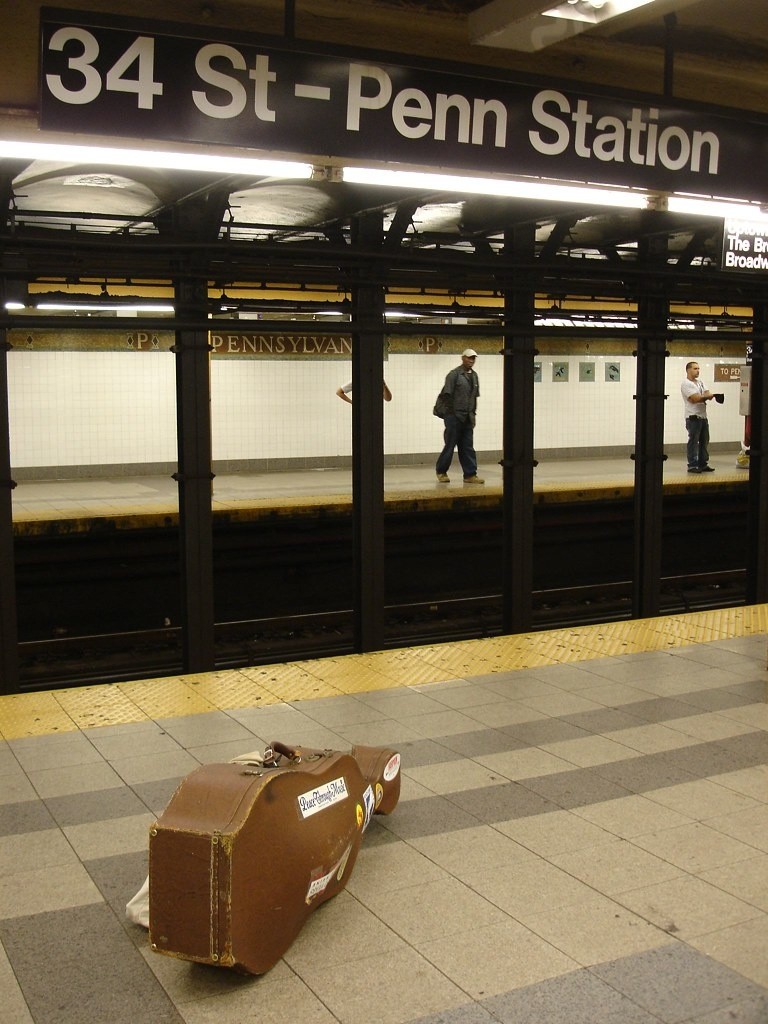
[698,466,714,471]
[436,472,450,482]
[464,476,484,484]
[688,468,702,473]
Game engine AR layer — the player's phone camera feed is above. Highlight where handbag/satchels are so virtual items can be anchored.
[433,369,458,419]
[736,453,749,468]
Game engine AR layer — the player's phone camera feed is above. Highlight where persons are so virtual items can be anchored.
[337,378,393,404]
[681,362,716,473]
[436,348,484,484]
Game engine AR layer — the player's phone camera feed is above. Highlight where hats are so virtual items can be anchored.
[714,394,724,403]
[463,349,478,357]
